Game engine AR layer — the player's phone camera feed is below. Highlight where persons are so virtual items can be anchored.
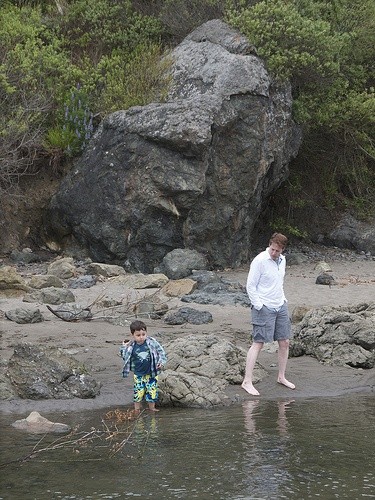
[120,320,167,413]
[240,233,296,396]
